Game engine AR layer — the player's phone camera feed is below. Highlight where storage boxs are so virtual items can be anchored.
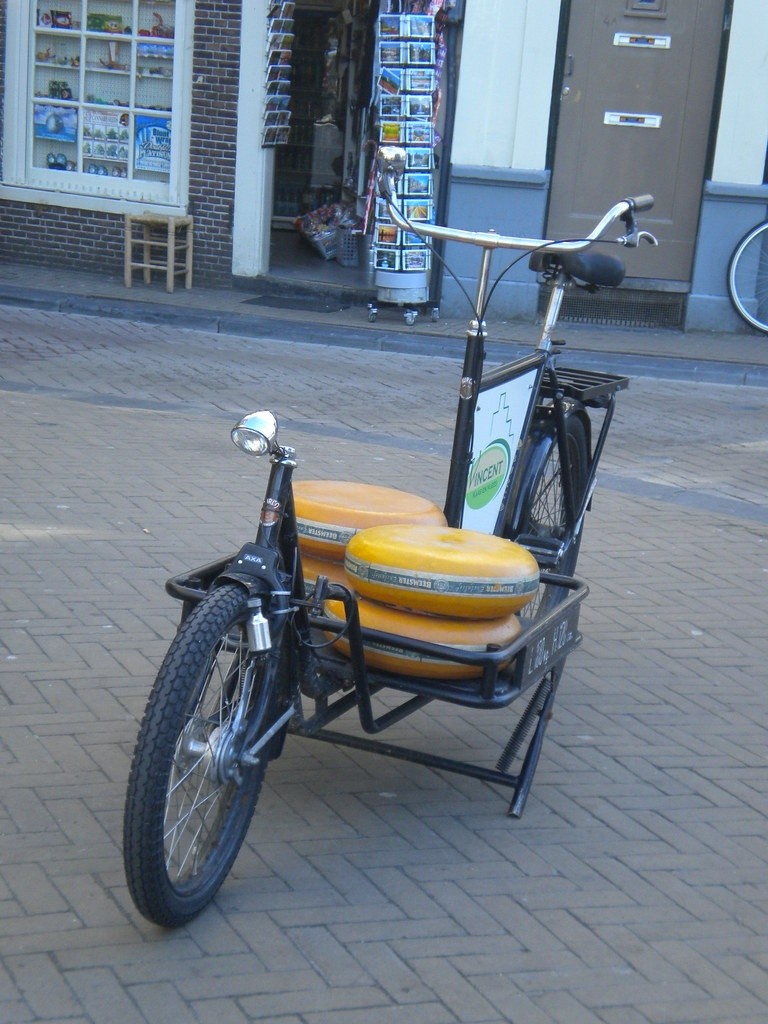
[335,228,361,266]
[300,208,337,259]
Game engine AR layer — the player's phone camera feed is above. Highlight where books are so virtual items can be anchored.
[263,0,295,144]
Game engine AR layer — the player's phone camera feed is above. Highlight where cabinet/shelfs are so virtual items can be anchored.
[271,0,386,232]
[25,0,176,185]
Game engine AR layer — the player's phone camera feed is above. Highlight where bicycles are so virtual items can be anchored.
[726,220,768,334]
[108,189,656,927]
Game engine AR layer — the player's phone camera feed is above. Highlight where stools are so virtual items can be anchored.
[125,210,194,294]
